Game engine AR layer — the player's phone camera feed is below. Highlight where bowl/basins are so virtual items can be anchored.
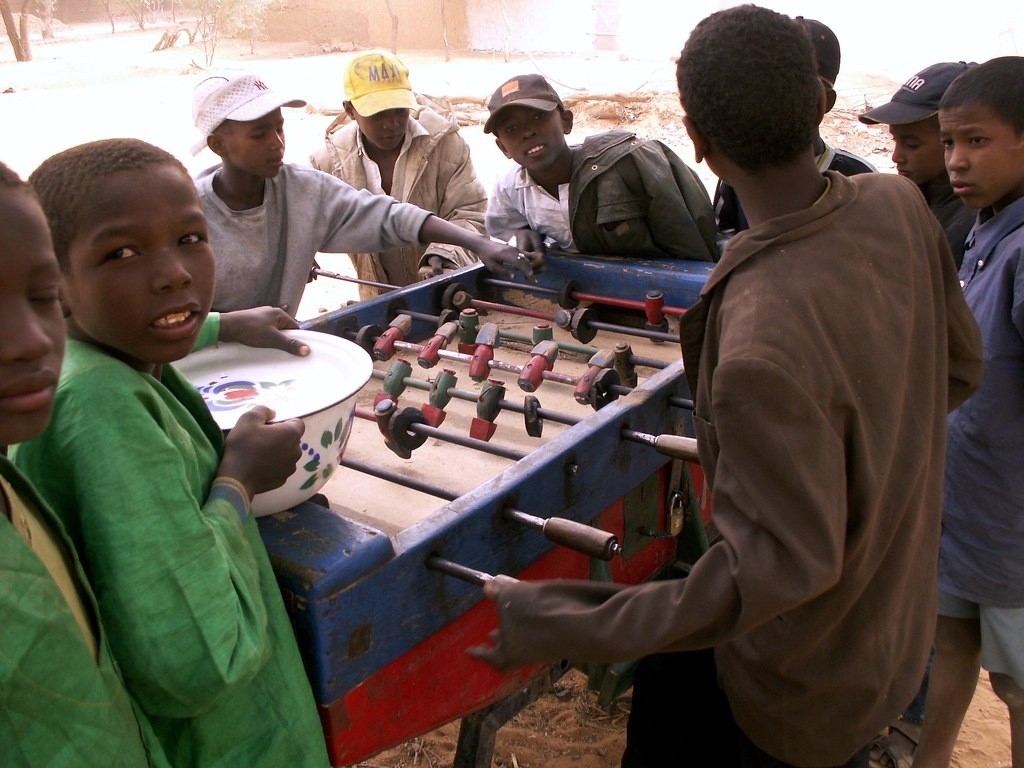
[173,329,372,517]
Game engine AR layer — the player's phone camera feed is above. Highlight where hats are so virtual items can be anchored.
[192,73,306,138]
[342,49,418,118]
[859,60,979,125]
[482,74,564,134]
[792,15,841,87]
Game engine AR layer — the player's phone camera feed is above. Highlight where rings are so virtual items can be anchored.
[515,252,527,261]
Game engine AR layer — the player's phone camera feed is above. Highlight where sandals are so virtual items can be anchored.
[867,718,919,768]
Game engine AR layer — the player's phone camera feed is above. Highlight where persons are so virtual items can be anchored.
[0,160,180,768]
[302,47,486,303]
[911,55,1024,768]
[16,140,317,767]
[486,1,986,768]
[857,61,976,767]
[174,70,542,327]
[487,75,722,265]
